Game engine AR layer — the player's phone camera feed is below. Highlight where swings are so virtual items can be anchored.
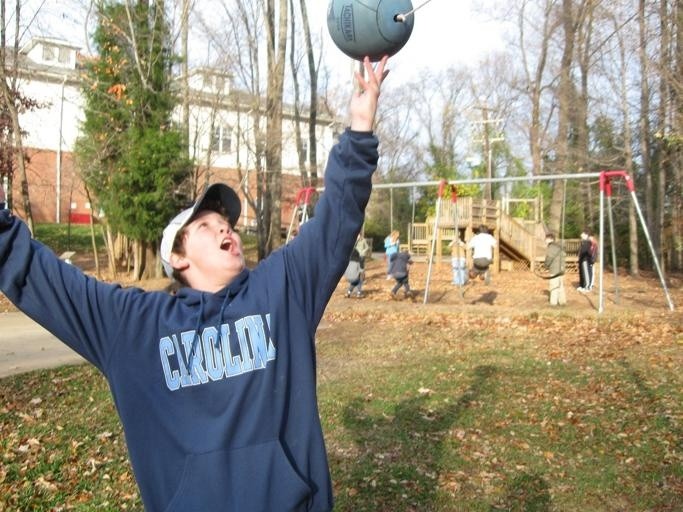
[533,179,567,280]
[467,184,504,272]
[390,187,415,283]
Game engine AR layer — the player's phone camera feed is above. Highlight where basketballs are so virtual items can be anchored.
[327,0,413,62]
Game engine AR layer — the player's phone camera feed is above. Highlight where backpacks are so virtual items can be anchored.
[587,237,599,264]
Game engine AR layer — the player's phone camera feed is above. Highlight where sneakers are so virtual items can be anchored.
[345,289,411,300]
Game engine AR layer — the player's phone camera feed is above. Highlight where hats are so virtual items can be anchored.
[156,182,243,281]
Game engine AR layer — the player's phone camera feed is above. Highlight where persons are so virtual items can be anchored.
[354,233,368,268]
[464,224,499,281]
[383,230,399,280]
[589,234,598,288]
[389,248,412,297]
[447,230,468,285]
[345,249,364,298]
[576,240,583,289]
[544,234,566,305]
[1,49,392,511]
[578,230,592,293]
